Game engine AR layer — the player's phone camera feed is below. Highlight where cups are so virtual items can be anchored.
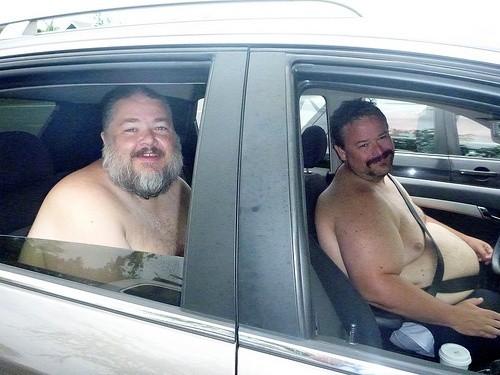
[439,343,471,370]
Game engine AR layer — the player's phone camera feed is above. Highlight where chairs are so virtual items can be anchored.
[0,131,57,237]
[302,126,327,245]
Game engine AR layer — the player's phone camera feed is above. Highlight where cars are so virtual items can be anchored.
[0,17,500,375]
[302,97,499,189]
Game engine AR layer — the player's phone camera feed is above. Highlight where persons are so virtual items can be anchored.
[315,99,500,372]
[17,85,191,307]
